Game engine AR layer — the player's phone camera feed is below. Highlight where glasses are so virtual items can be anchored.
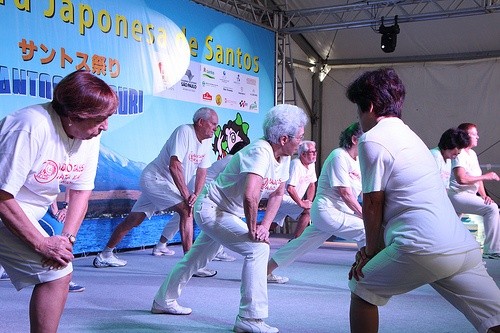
[303,151,317,153]
[288,135,304,141]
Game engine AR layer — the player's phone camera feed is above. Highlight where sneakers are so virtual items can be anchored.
[69,281,85,292]
[0,273,10,280]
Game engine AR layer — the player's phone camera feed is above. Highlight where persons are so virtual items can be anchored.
[0,66,500,333]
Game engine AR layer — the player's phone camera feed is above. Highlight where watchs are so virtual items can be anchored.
[62,233,76,244]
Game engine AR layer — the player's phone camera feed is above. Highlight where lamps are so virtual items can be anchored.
[380,14,401,52]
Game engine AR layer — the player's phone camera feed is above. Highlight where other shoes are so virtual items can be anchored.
[192,267,217,277]
[153,246,176,256]
[212,250,236,261]
[482,252,500,259]
[267,274,289,283]
[233,314,279,333]
[93,251,127,267]
[152,300,192,314]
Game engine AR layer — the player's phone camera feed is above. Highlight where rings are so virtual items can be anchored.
[352,263,357,267]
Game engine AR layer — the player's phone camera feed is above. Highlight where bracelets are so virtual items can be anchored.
[55,211,60,219]
[64,205,69,209]
